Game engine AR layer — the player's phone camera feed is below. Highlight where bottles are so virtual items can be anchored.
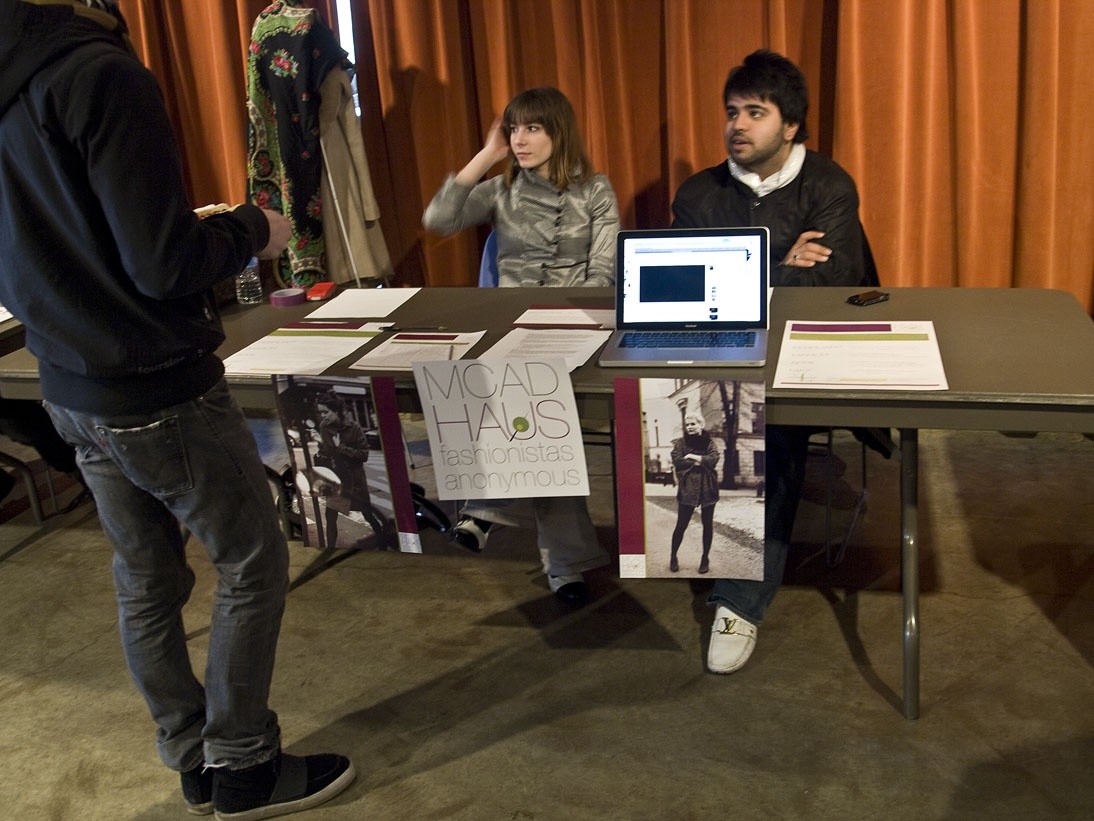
[236,258,264,305]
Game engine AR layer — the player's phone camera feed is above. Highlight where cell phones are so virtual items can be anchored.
[848,290,890,306]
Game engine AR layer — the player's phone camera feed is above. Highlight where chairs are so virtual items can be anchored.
[454,227,618,531]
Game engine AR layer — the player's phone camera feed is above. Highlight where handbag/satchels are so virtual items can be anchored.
[312,452,354,497]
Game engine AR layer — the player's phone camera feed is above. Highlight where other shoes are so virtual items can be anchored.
[699,556,709,573]
[671,558,677,572]
[449,515,494,556]
[547,573,588,606]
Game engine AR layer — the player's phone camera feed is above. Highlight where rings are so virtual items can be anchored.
[794,254,799,260]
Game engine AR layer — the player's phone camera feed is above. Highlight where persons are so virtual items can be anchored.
[670,413,719,574]
[672,48,895,673]
[421,87,620,603]
[310,389,384,549]
[0,0,355,821]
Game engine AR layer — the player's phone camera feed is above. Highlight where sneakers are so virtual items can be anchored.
[211,751,356,821]
[707,604,758,674]
[179,759,214,816]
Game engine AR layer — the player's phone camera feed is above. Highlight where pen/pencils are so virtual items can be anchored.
[384,326,445,330]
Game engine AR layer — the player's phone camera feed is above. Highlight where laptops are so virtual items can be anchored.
[597,226,771,369]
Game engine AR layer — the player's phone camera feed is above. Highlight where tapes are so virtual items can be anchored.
[270,288,305,306]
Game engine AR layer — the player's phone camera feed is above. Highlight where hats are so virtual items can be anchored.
[279,464,292,477]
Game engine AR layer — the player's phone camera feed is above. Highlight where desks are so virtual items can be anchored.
[0,288,1094,718]
